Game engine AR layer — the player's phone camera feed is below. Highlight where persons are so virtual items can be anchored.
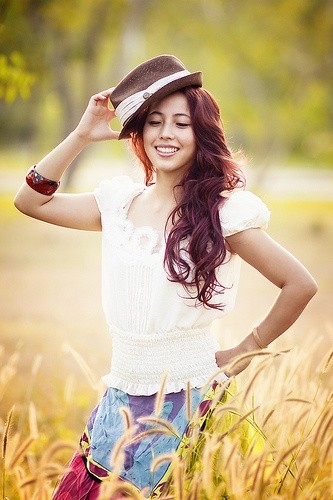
[12,53,319,500]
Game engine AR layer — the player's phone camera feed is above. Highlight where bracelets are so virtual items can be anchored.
[23,165,62,195]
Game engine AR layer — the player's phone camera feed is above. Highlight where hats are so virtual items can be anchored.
[109,55,202,141]
